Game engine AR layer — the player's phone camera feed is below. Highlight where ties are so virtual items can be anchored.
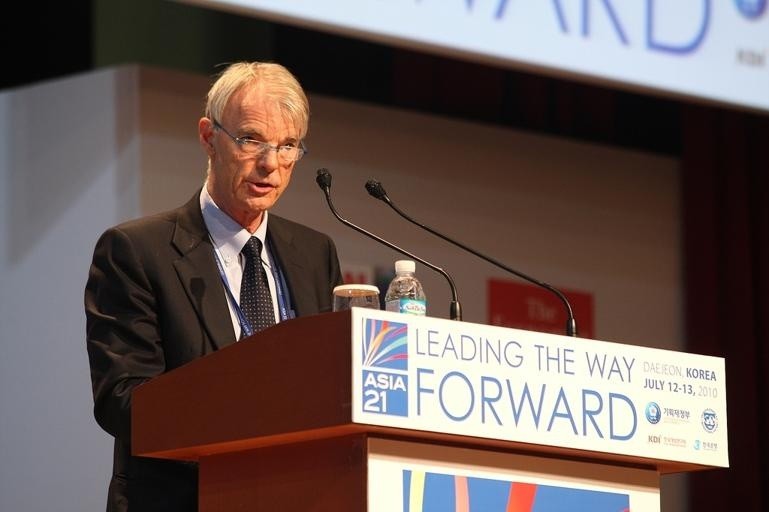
[240,237,276,340]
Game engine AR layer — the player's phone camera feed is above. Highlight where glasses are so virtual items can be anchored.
[214,119,307,161]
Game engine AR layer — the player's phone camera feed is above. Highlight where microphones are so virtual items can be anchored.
[315,167,462,320]
[364,177,576,338]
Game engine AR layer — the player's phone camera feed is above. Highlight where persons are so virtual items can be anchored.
[83,61,343,512]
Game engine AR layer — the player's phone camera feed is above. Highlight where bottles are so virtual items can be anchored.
[384,259,426,315]
[332,283,381,311]
